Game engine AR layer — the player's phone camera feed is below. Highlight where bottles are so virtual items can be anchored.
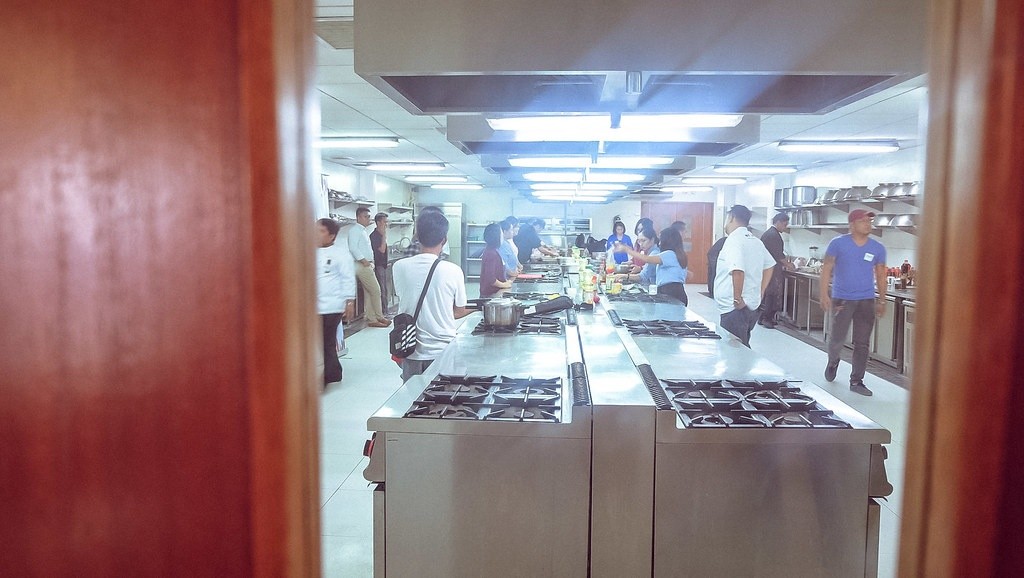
[886,267,891,276]
[895,267,901,278]
[578,260,587,287]
[572,243,576,258]
[600,255,606,281]
[891,267,895,276]
[901,259,912,286]
[583,266,594,304]
[606,275,615,294]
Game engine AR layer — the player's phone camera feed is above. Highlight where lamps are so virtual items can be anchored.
[367,163,445,170]
[404,175,468,182]
[484,111,745,168]
[321,135,399,149]
[659,186,714,192]
[778,138,900,152]
[681,176,746,185]
[432,183,485,190]
[712,164,799,174]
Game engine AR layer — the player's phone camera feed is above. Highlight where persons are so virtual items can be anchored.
[614,218,688,307]
[713,203,777,349]
[500,216,523,279]
[707,237,727,296]
[818,209,887,396]
[480,224,513,295]
[760,212,795,328]
[514,219,559,263]
[314,218,356,384]
[347,206,396,327]
[403,206,449,260]
[607,222,633,264]
[391,210,479,385]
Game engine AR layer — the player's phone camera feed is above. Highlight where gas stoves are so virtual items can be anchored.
[502,292,553,300]
[521,268,560,272]
[471,316,562,336]
[513,277,558,283]
[622,318,721,338]
[607,293,676,303]
[363,373,563,482]
[659,378,894,497]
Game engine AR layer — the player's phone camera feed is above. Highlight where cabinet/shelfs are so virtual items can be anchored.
[328,197,376,223]
[378,202,415,228]
[464,222,493,281]
[774,193,921,238]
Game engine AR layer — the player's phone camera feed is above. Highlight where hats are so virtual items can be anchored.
[849,209,876,222]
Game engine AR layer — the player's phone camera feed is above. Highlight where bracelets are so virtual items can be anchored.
[878,301,887,305]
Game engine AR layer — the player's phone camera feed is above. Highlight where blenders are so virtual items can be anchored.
[808,245,818,267]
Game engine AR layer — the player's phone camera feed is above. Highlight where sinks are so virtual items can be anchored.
[402,249,418,255]
[795,267,814,275]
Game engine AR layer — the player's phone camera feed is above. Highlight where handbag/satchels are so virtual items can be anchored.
[390,313,417,357]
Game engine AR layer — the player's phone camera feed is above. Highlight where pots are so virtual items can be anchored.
[791,209,819,225]
[870,181,924,197]
[873,214,917,227]
[783,187,792,207]
[775,189,782,207]
[820,186,871,201]
[465,296,522,326]
[792,186,816,206]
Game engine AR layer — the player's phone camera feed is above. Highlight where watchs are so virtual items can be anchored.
[734,297,743,306]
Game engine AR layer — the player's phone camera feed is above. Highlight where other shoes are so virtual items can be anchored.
[369,319,387,327]
[378,317,392,325]
[851,381,873,396]
[762,319,773,328]
[825,360,839,381]
[758,320,777,325]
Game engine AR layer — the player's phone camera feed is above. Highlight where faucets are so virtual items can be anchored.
[398,237,410,252]
[805,257,817,266]
[391,240,400,254]
